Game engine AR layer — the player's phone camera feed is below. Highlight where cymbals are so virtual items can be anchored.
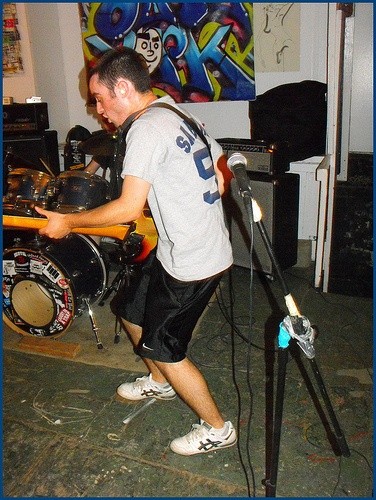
[77,130,120,157]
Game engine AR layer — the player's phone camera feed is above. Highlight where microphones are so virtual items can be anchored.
[226,152,253,200]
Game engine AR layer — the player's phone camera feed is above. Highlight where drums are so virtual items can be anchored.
[2,234,109,338]
[49,171,113,213]
[2,167,56,218]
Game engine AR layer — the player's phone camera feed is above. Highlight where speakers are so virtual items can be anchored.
[4,131,59,178]
[214,172,299,279]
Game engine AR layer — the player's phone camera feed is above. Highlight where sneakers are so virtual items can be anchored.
[116,373,177,401]
[170,418,238,455]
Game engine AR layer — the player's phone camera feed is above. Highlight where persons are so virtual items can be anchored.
[34,46,238,456]
[83,114,117,174]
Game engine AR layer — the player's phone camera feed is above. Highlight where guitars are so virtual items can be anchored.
[2,213,159,264]
